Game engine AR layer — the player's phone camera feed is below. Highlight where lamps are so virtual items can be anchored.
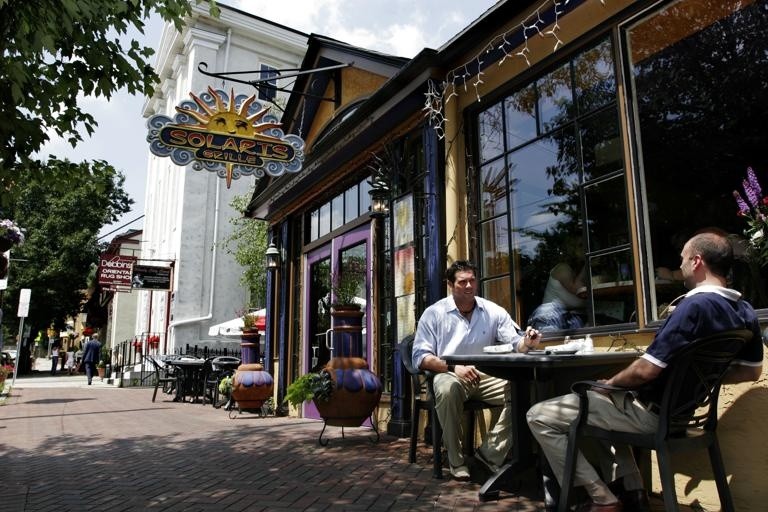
[265,234,281,273]
[366,168,392,220]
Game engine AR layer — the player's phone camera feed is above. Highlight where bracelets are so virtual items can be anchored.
[523,334,535,351]
[447,363,456,373]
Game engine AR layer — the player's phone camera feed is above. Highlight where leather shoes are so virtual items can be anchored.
[88,381,91,385]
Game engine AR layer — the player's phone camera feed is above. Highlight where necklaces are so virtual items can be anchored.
[459,305,475,315]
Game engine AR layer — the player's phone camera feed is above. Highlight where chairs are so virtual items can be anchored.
[143,350,243,408]
[397,333,508,480]
[551,329,755,511]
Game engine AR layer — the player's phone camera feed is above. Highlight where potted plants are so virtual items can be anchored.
[320,257,369,310]
[95,358,107,378]
[240,313,259,334]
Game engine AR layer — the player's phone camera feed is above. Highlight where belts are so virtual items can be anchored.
[590,489,649,511]
[450,464,470,482]
[474,452,500,473]
[638,395,661,415]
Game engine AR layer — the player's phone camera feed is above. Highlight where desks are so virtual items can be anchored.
[439,346,642,502]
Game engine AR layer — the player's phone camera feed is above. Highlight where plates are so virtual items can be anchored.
[552,347,579,355]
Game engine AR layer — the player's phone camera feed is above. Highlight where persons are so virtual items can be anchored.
[80,333,102,384]
[51,345,83,375]
[528,233,601,330]
[410,260,543,479]
